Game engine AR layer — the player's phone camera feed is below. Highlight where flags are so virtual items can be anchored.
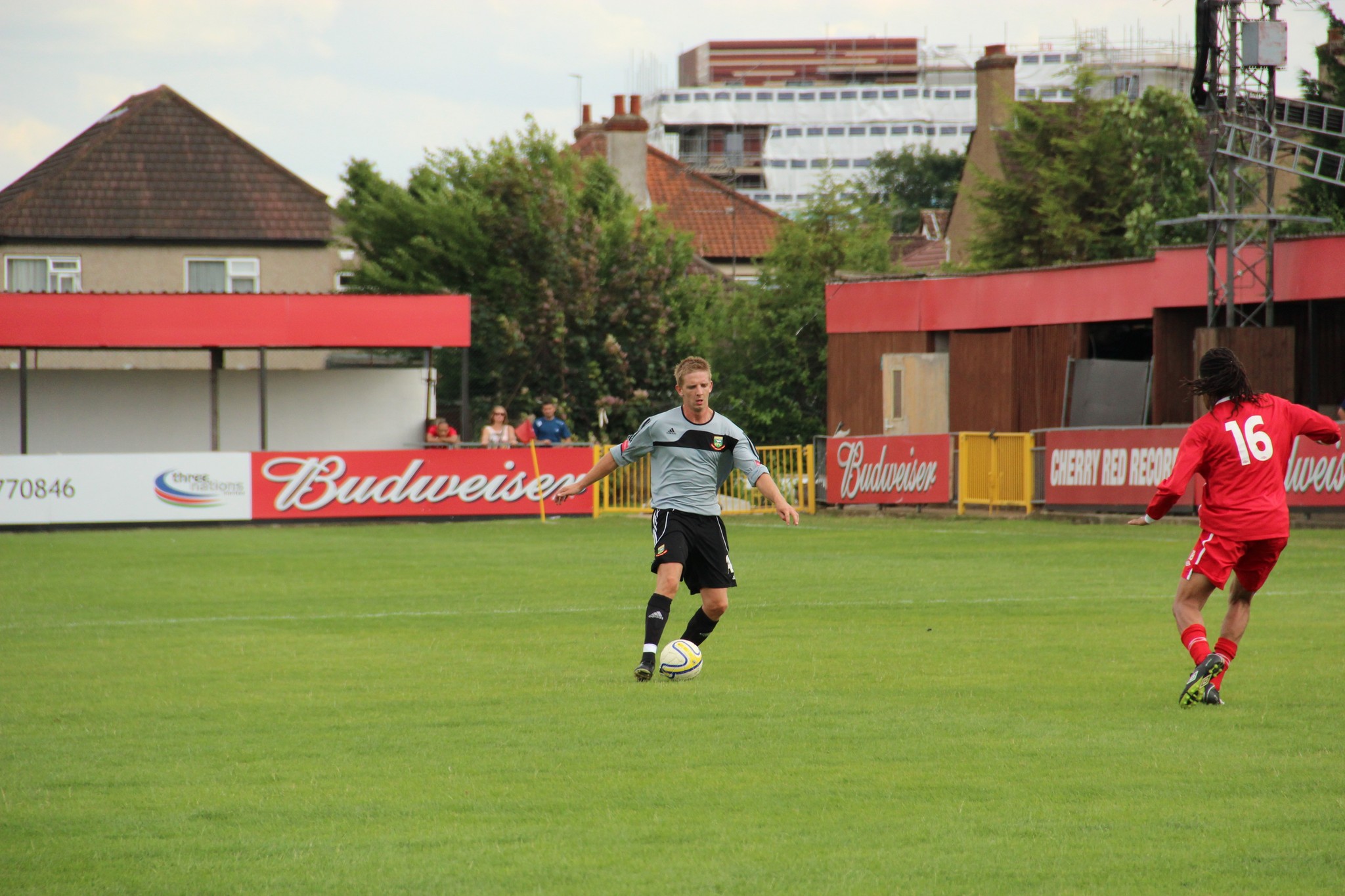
[516,420,536,445]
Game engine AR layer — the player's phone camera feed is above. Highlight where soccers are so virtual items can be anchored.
[660,640,704,682]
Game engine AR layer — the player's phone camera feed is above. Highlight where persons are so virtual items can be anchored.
[1127,347,1342,709]
[552,356,800,682]
[426,421,458,449]
[532,403,572,447]
[480,406,519,449]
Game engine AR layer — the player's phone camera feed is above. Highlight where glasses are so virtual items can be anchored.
[494,412,504,416]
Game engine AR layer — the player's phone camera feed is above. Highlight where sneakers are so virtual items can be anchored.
[634,661,653,682]
[1178,654,1225,709]
[1200,682,1220,704]
